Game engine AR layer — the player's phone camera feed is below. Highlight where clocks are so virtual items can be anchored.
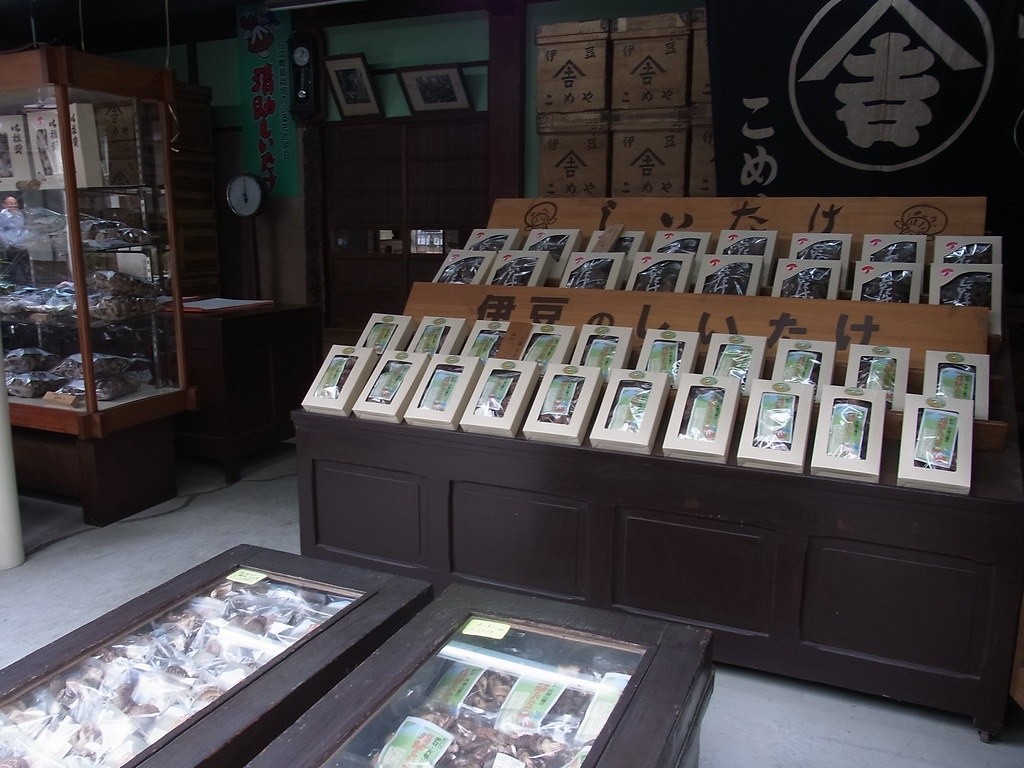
[287,27,327,125]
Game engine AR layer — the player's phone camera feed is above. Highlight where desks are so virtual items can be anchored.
[158,297,322,484]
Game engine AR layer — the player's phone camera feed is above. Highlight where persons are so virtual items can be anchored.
[0,194,32,287]
[417,74,458,105]
[344,73,359,98]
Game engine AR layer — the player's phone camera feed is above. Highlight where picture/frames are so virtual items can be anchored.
[398,68,474,116]
[323,53,384,122]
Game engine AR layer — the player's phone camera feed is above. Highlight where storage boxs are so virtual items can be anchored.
[536,9,723,197]
[302,225,1004,494]
[0,100,220,289]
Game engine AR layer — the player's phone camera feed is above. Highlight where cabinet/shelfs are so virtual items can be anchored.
[0,50,193,435]
